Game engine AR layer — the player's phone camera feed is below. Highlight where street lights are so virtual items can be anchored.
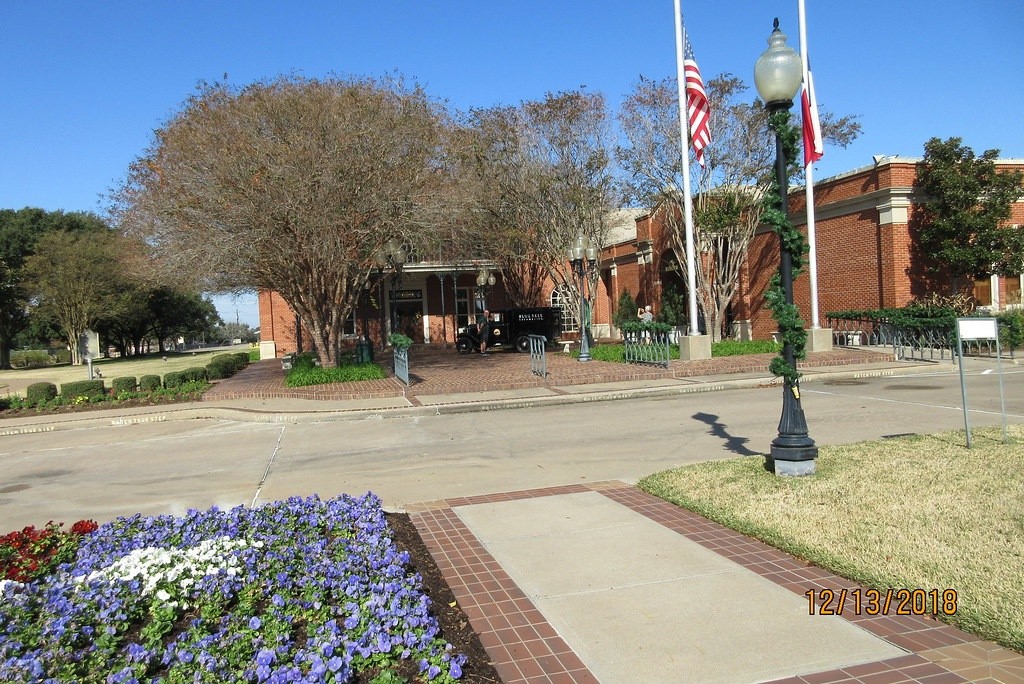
[374,237,407,335]
[476,265,496,313]
[567,228,598,362]
[362,277,373,344]
[752,14,819,464]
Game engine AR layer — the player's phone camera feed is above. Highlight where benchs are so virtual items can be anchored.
[557,339,598,353]
[280,352,296,371]
[832,331,862,346]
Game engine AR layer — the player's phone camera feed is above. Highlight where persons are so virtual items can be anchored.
[477,310,493,357]
[637,305,653,323]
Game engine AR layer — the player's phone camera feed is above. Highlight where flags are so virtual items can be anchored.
[681,15,712,170]
[799,50,824,170]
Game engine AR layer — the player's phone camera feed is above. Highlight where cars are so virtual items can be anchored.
[456,307,562,355]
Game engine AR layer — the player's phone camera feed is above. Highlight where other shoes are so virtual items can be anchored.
[480,353,490,357]
